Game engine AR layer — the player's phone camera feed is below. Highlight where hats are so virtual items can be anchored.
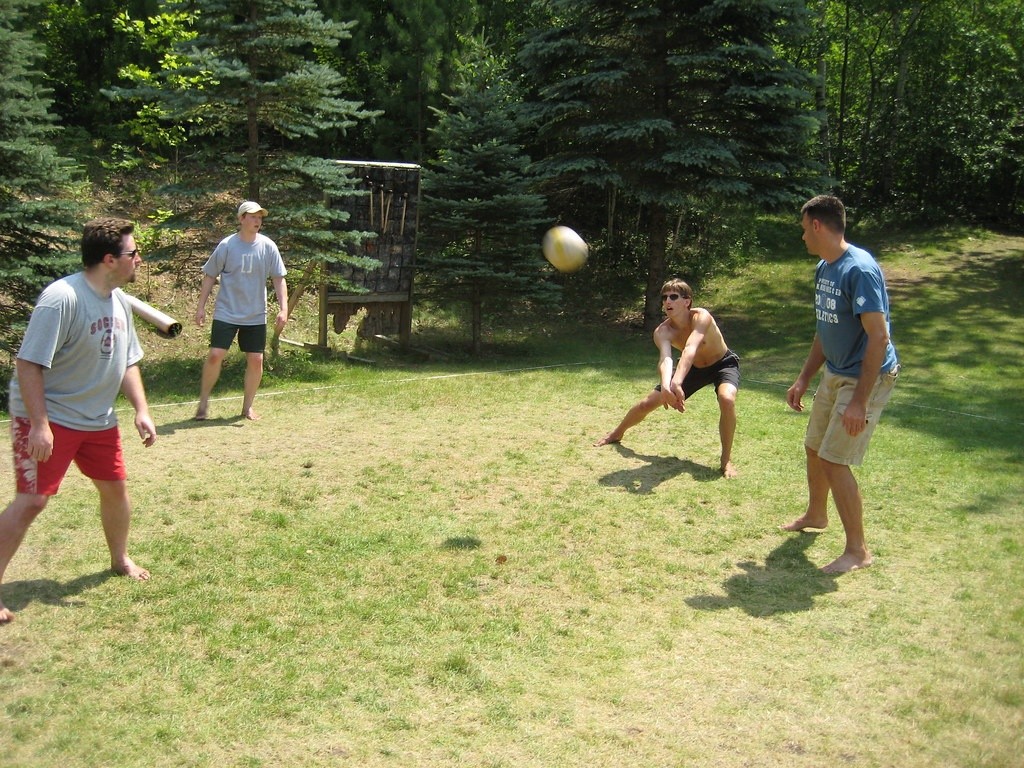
[237,201,268,218]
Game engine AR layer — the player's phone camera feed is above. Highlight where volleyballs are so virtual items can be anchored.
[543,225,588,272]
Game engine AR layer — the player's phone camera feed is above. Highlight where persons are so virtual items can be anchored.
[780,195,900,574]
[592,279,741,478]
[0,217,156,621]
[194,201,288,420]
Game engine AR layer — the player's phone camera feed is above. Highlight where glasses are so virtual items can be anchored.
[120,249,136,259]
[662,294,678,300]
